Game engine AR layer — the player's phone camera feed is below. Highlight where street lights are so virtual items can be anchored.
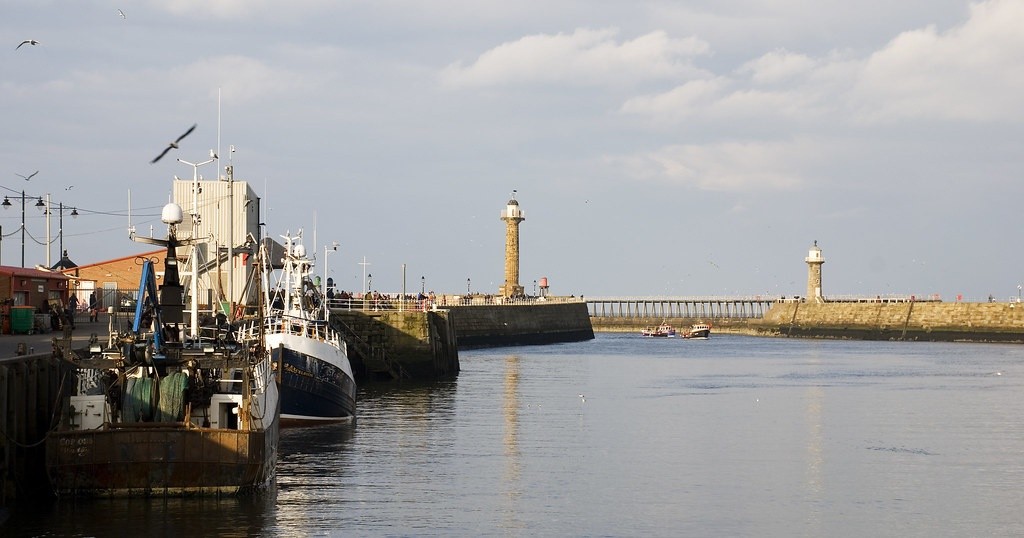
[421,276,425,295]
[533,279,537,298]
[0,189,46,269]
[367,273,372,292]
[402,263,406,300]
[43,202,79,272]
[466,278,470,295]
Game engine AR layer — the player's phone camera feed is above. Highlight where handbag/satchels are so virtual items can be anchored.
[91,309,98,316]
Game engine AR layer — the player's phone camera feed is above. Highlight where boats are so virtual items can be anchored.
[641,324,675,338]
[681,322,711,339]
[45,144,357,500]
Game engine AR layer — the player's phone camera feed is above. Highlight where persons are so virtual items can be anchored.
[571,295,574,298]
[463,293,529,305]
[43,300,52,314]
[781,296,785,303]
[69,292,80,315]
[988,294,994,303]
[580,295,583,301]
[89,291,99,322]
[263,284,446,311]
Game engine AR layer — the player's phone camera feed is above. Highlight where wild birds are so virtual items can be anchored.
[333,241,340,246]
[13,170,40,182]
[65,185,74,190]
[117,8,126,20]
[209,149,218,159]
[15,40,39,51]
[150,124,196,163]
